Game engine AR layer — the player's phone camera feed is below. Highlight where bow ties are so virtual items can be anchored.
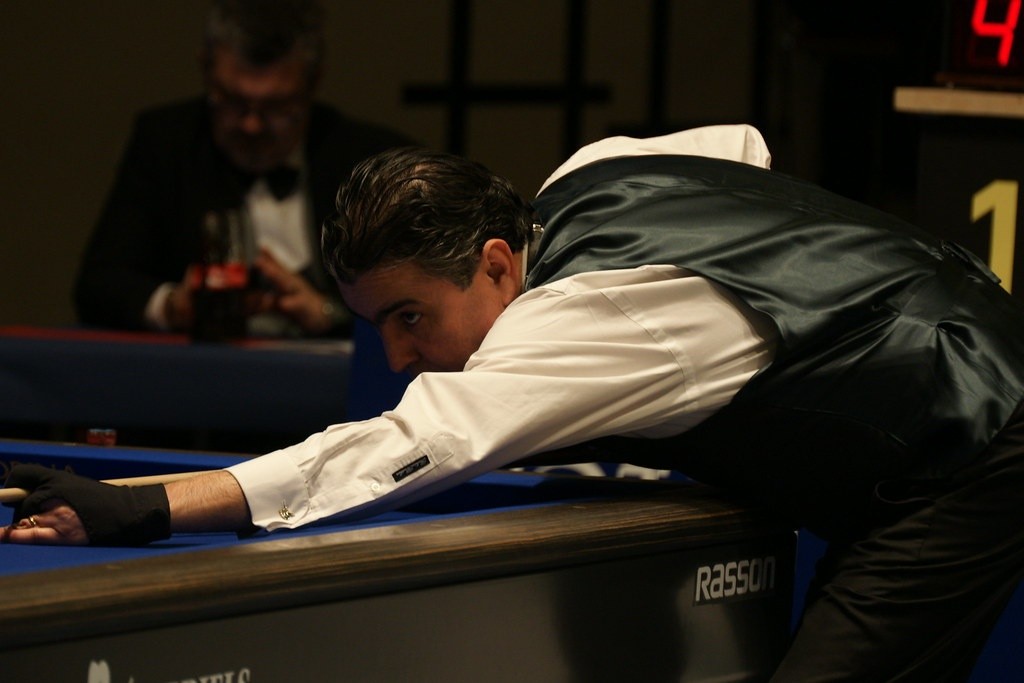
[234,161,302,203]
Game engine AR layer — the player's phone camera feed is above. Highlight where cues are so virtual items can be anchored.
[0,469,223,513]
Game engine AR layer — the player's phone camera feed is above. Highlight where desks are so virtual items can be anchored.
[0,444,735,683]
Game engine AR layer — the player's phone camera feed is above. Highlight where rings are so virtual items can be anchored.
[28,514,38,528]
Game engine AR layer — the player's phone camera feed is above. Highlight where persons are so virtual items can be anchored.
[0,122,1024,683]
[69,0,418,352]
